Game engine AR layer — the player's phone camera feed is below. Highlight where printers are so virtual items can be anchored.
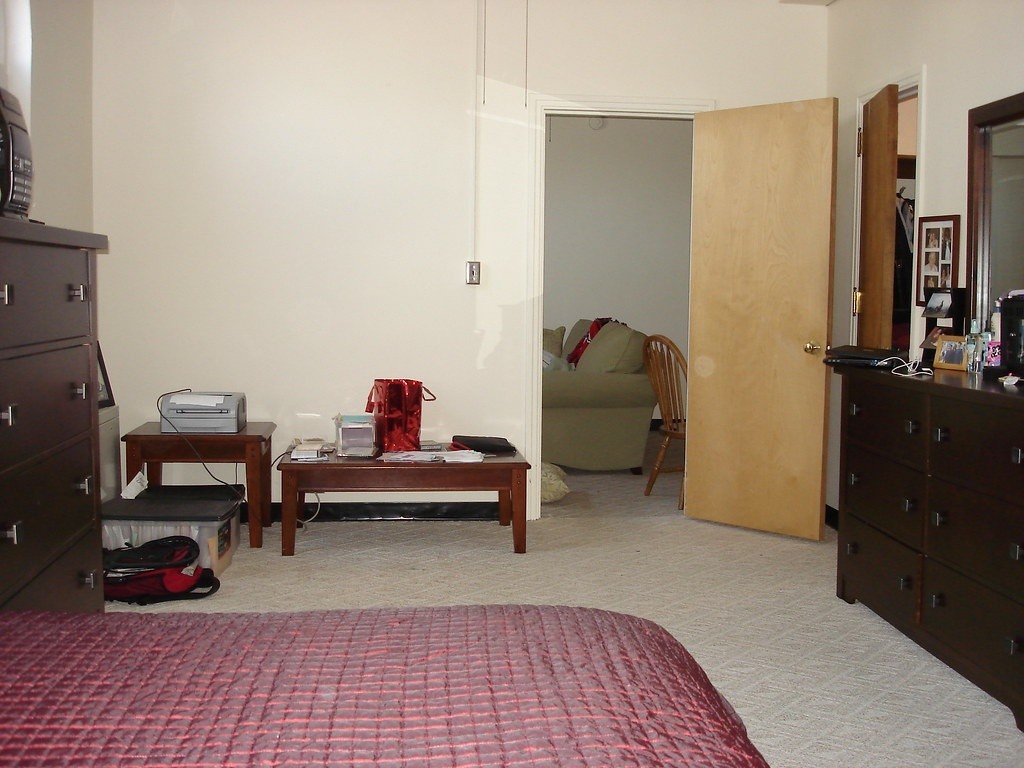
[160,391,248,433]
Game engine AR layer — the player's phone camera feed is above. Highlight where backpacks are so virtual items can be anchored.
[102,534,220,605]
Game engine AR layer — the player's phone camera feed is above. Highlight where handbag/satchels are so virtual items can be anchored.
[364,379,434,451]
[448,436,517,454]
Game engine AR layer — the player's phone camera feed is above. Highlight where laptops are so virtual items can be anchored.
[823,345,908,366]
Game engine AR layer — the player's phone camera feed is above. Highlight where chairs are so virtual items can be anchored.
[642,334,687,511]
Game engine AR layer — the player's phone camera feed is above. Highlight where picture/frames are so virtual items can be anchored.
[97,340,116,409]
[916,215,961,307]
[933,334,967,371]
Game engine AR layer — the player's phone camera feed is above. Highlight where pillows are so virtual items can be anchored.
[543,326,566,358]
[562,318,592,353]
[575,322,648,373]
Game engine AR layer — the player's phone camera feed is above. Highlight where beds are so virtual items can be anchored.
[0,611,770,768]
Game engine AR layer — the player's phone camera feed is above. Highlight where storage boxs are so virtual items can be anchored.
[102,484,246,576]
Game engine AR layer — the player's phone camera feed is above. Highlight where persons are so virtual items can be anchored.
[924,227,963,365]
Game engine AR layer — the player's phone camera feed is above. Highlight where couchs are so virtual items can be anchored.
[542,319,662,475]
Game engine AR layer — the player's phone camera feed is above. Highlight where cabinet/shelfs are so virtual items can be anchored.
[833,361,1024,733]
[0,216,109,613]
[99,405,122,503]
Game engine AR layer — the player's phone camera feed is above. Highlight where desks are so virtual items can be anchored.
[277,442,532,556]
[120,421,278,548]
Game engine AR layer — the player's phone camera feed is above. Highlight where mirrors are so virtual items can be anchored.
[965,92,1024,335]
